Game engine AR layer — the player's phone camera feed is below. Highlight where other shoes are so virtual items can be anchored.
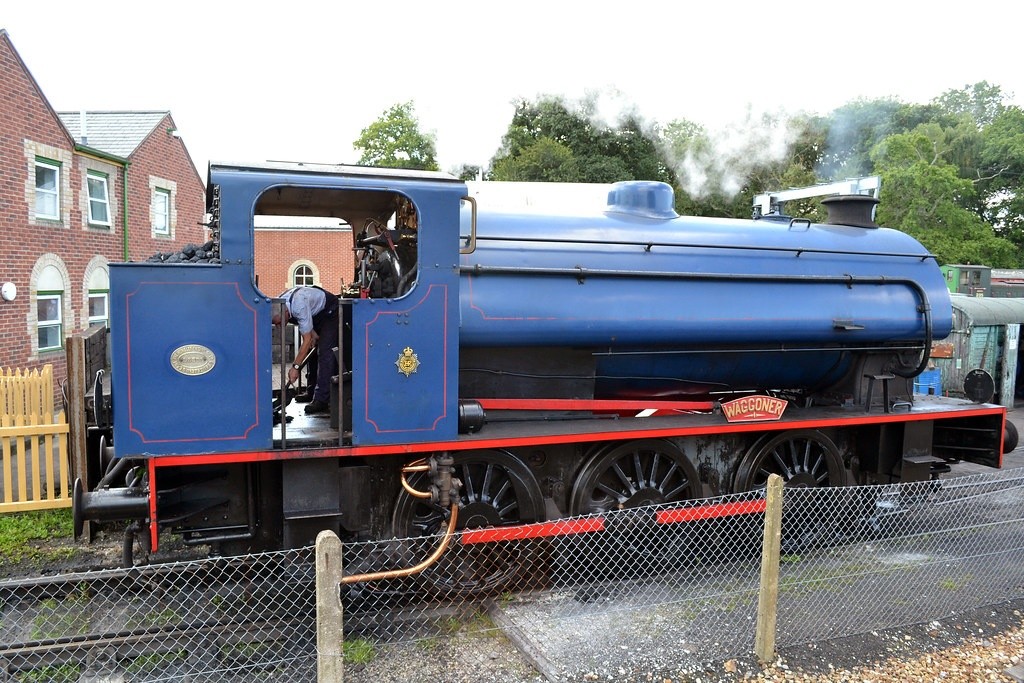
[304,399,328,412]
[295,390,314,402]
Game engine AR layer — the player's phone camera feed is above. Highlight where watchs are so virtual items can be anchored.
[292,363,301,370]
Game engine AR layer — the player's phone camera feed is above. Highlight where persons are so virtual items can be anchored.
[272,285,339,414]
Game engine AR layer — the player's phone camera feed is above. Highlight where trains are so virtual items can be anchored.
[72,158,1019,604]
